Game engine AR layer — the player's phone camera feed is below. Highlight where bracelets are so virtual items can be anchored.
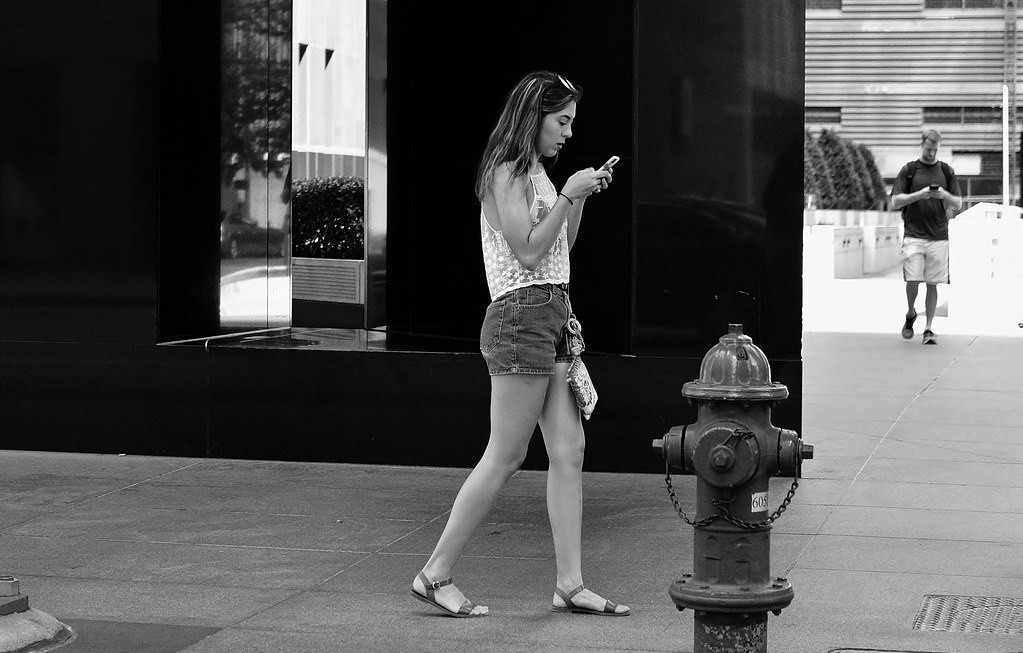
[559,192,574,206]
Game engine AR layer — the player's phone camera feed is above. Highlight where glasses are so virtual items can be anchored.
[544,75,576,97]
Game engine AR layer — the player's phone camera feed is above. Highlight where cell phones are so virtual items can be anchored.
[930,184,940,192]
[597,155,620,171]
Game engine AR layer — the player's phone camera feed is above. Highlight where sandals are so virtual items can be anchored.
[411,571,488,618]
[552,584,630,616]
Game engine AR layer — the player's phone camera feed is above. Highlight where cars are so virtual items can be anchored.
[221,213,266,259]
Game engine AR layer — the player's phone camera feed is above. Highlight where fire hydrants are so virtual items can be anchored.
[653,324,814,653]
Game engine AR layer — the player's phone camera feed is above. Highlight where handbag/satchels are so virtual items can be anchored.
[566,357,598,420]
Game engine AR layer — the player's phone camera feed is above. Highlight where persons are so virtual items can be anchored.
[888,130,963,345]
[412,72,633,615]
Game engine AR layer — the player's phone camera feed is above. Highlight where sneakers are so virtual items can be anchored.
[902,310,917,338]
[922,329,936,344]
